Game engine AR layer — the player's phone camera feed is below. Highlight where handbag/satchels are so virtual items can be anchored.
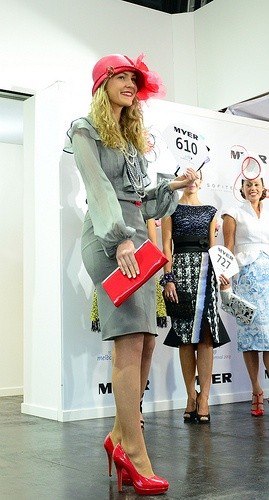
[221,294,257,326]
[101,239,169,308]
[163,290,193,318]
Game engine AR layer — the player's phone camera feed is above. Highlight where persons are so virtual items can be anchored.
[63,54,169,495]
[161,166,232,422]
[219,177,269,416]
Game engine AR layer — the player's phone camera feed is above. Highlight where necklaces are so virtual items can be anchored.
[119,143,146,198]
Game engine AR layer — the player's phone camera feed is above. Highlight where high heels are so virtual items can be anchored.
[104,433,134,486]
[251,391,264,417]
[197,392,210,423]
[112,442,169,495]
[184,389,198,421]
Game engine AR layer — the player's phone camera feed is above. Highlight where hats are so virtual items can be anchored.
[91,53,160,100]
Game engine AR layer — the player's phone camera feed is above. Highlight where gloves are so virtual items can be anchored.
[220,286,233,304]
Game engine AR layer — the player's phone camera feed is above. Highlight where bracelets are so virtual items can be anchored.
[161,273,174,284]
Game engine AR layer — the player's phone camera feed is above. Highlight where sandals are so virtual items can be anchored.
[139,408,144,428]
